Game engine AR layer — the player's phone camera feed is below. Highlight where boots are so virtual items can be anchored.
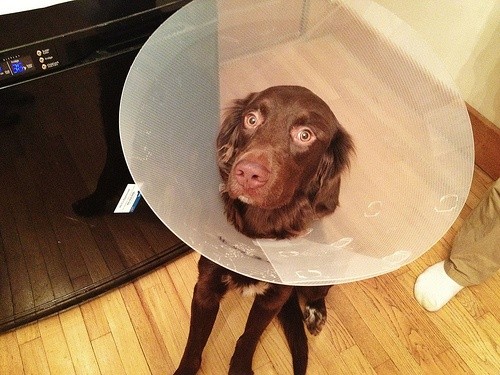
[72,60,145,219]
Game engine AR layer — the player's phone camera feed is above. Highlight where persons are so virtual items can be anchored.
[412,172,500,313]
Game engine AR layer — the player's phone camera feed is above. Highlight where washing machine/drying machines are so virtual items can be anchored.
[0,0,222,335]
